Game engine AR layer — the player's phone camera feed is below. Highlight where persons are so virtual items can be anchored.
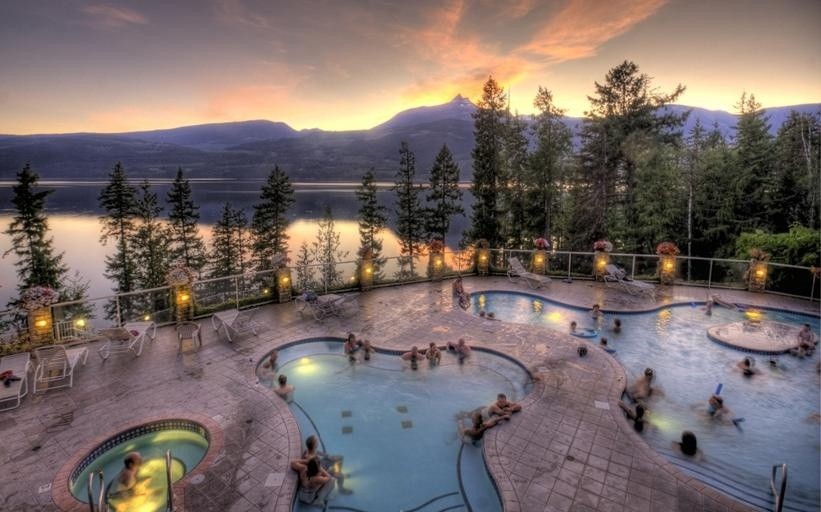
[269,373,296,397]
[258,360,277,382]
[342,275,821,462]
[303,435,354,495]
[108,449,162,500]
[267,349,282,368]
[289,456,335,510]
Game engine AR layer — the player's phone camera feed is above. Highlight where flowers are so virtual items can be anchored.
[356,245,377,259]
[658,242,681,255]
[162,264,194,283]
[592,240,615,253]
[16,285,59,310]
[750,246,773,263]
[272,249,293,270]
[535,239,549,248]
[428,238,442,254]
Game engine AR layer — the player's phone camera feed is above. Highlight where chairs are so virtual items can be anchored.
[294,290,361,325]
[178,321,202,348]
[212,309,264,343]
[30,338,90,390]
[1,346,35,412]
[92,319,158,361]
[600,264,655,300]
[505,257,551,289]
